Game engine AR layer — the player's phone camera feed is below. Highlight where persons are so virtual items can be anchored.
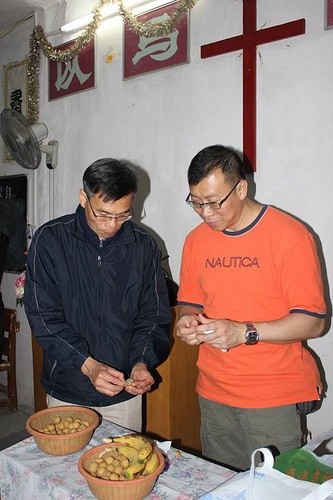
[175,146,328,473]
[24,158,173,433]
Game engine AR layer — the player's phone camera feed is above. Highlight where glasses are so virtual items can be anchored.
[185,181,240,210]
[85,191,134,222]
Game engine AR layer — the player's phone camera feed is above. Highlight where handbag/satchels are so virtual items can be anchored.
[198,448,333,500]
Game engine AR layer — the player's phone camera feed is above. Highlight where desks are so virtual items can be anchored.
[0,418,239,500]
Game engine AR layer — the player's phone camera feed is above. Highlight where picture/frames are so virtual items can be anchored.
[122,0,190,82]
[4,59,29,161]
[47,33,95,102]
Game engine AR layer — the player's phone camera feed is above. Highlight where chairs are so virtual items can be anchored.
[0,308,18,413]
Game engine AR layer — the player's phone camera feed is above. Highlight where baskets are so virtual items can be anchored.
[26,407,99,456]
[78,442,165,500]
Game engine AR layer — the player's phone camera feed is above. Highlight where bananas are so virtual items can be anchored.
[103,436,159,480]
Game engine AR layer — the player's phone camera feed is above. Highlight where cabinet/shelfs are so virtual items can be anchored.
[31,305,203,455]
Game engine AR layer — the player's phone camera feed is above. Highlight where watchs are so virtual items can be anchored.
[245,322,259,345]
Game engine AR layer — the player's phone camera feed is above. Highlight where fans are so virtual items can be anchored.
[0,108,58,170]
